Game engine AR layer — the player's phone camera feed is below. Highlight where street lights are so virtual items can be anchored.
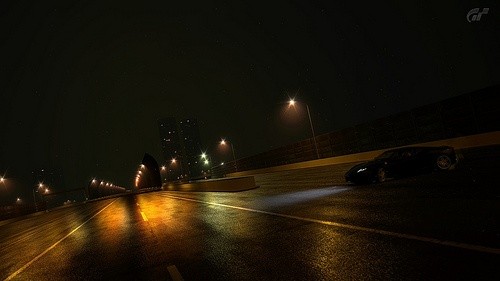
[220,138,240,172]
[0,149,214,226]
[288,99,321,159]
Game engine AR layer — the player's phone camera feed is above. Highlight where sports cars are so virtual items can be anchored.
[343,146,457,185]
